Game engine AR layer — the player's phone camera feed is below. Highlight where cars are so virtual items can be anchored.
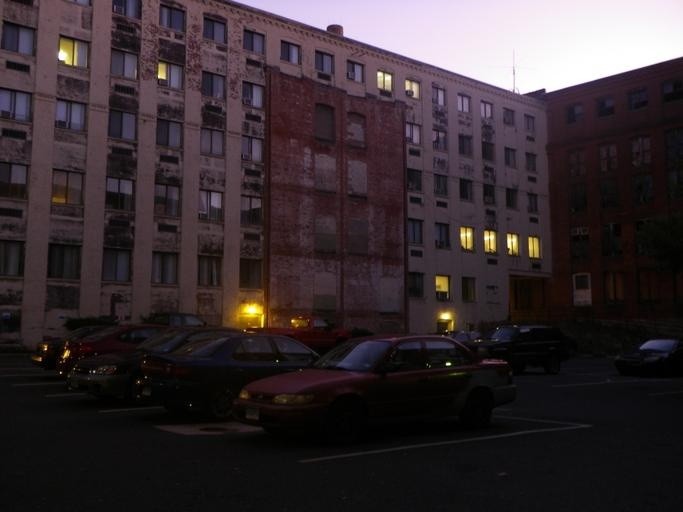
[28,323,324,424]
[612,336,682,379]
[230,334,519,447]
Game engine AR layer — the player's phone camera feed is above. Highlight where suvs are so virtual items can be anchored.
[459,323,563,374]
